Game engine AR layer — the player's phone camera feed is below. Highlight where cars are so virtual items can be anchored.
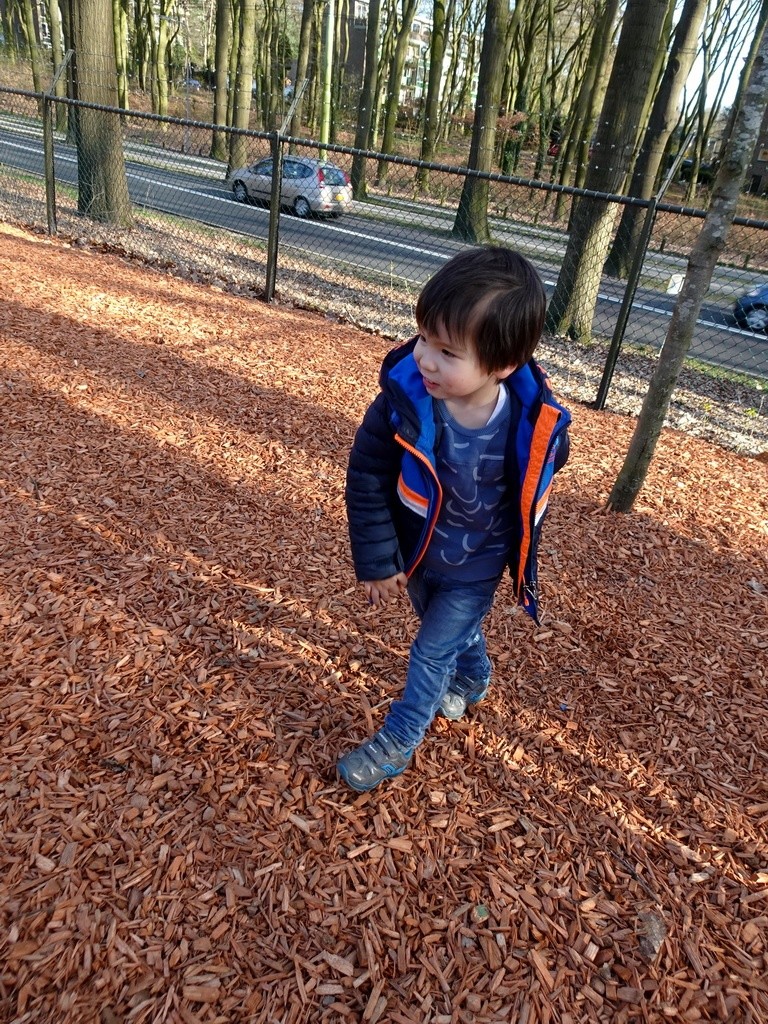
[732,283,768,335]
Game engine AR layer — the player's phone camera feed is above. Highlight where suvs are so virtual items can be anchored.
[227,155,354,220]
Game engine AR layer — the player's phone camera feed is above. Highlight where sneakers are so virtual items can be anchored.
[336,729,414,791]
[435,670,490,719]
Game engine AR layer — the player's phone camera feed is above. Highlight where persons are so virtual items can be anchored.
[336,241,571,791]
